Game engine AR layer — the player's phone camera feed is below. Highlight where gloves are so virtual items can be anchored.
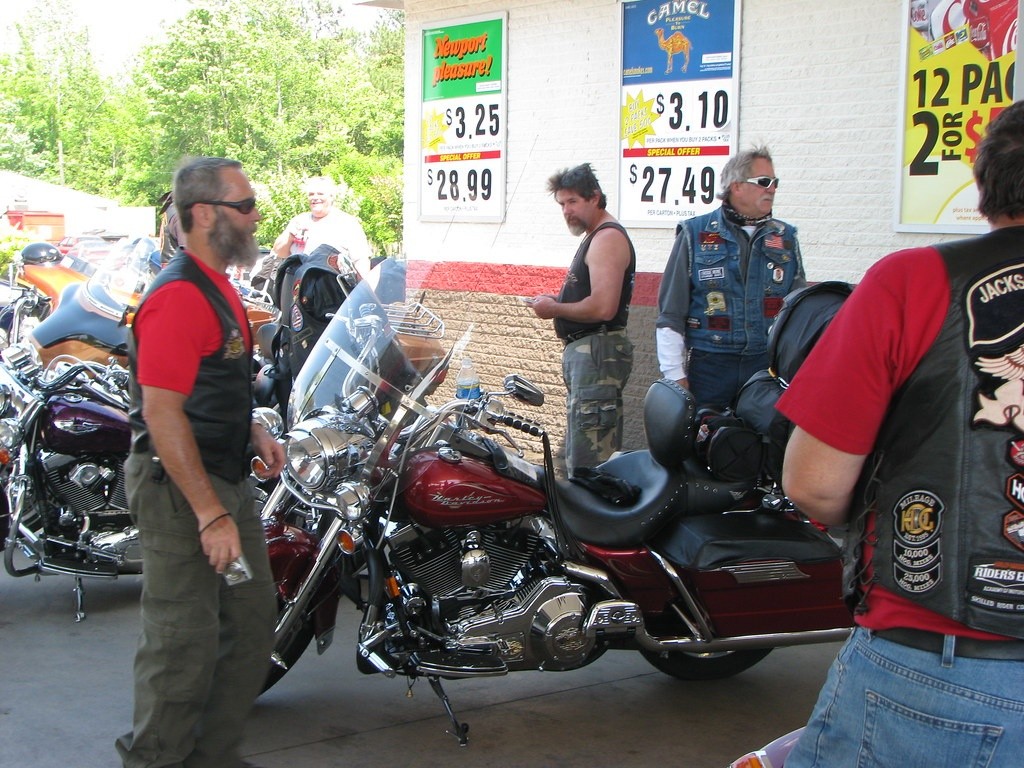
[567,465,643,509]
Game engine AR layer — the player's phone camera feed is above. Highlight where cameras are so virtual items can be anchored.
[223,554,253,586]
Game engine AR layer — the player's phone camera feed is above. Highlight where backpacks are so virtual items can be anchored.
[271,244,416,439]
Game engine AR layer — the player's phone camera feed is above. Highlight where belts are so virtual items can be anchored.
[870,626,1024,660]
[564,324,624,345]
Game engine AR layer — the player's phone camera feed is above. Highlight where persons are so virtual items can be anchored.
[655,142,806,419]
[273,176,370,280]
[157,192,185,269]
[525,163,636,477]
[776,100,1024,768]
[115,158,286,768]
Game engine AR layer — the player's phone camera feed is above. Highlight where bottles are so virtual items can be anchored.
[455,356,480,429]
[290,213,316,255]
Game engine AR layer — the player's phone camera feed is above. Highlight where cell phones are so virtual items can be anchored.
[521,297,534,304]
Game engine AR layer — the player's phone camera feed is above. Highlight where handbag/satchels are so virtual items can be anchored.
[694,407,763,481]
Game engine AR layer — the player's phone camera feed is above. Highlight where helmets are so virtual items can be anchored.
[20,242,62,269]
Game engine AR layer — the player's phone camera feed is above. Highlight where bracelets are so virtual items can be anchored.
[199,512,230,535]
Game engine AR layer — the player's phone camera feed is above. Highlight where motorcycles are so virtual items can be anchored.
[0,228,453,623]
[256,253,856,749]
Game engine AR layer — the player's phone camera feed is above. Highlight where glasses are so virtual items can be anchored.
[184,198,255,214]
[737,176,779,189]
